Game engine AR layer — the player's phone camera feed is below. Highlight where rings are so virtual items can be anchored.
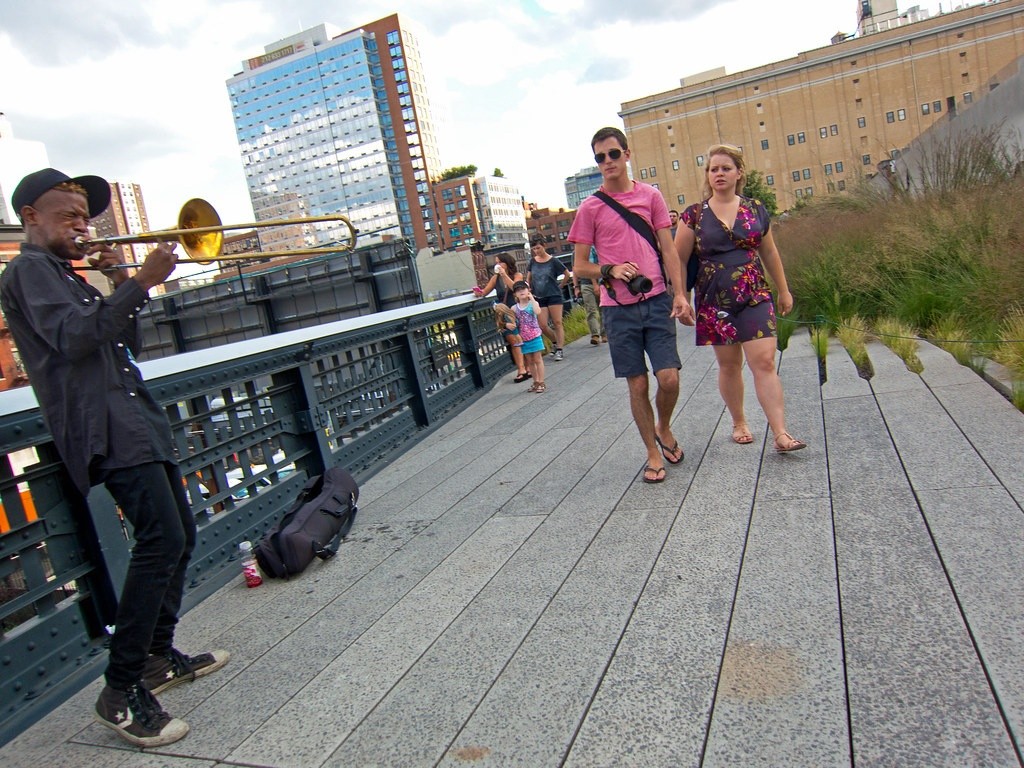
[625,272,629,275]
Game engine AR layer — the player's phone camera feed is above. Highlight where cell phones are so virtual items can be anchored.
[473,287,482,293]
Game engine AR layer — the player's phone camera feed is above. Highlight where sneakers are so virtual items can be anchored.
[554,350,564,361]
[140,647,231,696]
[590,336,600,345]
[94,682,191,746]
[550,344,557,356]
[602,336,608,342]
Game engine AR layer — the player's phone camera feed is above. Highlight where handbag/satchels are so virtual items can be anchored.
[497,320,507,336]
[687,202,702,289]
[253,467,359,580]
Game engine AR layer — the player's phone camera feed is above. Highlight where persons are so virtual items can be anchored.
[570,244,608,345]
[502,281,546,393]
[668,210,679,241]
[0,169,231,747]
[673,143,809,454]
[474,253,532,383]
[568,128,690,484]
[526,234,571,360]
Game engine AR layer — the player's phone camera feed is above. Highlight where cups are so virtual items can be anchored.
[494,265,501,273]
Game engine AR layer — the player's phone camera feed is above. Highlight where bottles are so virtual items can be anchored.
[239,541,263,589]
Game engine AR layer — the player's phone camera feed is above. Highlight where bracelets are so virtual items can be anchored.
[573,286,579,288]
[503,322,506,329]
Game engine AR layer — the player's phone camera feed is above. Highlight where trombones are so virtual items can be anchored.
[62,199,358,271]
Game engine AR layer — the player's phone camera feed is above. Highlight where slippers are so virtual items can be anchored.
[654,426,684,464]
[643,453,666,483]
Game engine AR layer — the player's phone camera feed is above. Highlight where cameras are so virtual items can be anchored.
[626,274,652,296]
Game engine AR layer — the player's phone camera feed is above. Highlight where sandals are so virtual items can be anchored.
[526,368,532,378]
[731,422,753,443]
[514,372,528,382]
[775,433,807,453]
[537,382,546,393]
[528,382,540,391]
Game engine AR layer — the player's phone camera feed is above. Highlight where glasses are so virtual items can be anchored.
[595,147,626,163]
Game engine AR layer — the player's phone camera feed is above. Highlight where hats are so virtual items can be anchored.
[512,281,528,291]
[13,167,113,218]
